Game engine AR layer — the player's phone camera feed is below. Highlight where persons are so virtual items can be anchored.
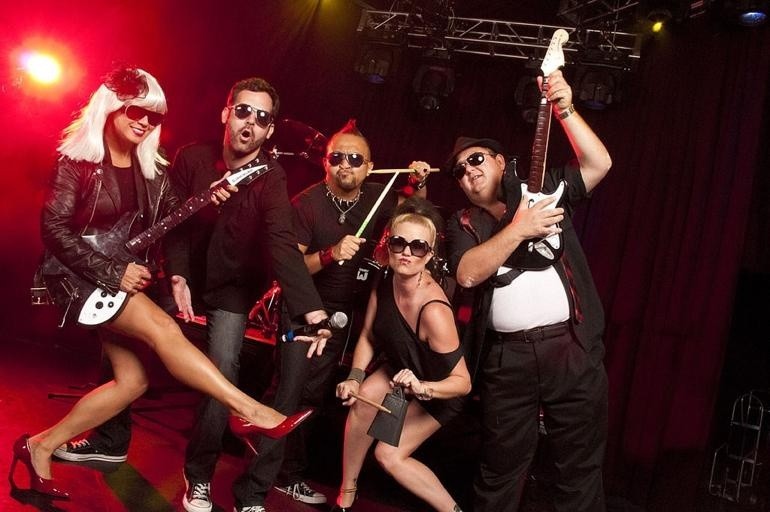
[8,66,314,499]
[50,78,332,512]
[445,69,612,511]
[231,118,430,512]
[333,212,472,512]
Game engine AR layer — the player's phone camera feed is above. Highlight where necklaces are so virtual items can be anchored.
[324,183,360,223]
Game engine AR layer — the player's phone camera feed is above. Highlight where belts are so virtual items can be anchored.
[494,320,572,342]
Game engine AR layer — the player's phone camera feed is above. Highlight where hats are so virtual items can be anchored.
[444,136,501,168]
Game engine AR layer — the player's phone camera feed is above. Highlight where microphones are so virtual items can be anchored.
[281,310,349,343]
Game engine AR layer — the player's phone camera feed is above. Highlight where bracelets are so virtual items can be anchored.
[411,180,427,190]
[556,105,578,120]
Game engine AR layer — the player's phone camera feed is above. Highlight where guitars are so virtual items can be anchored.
[490,25,571,273]
[47,148,279,329]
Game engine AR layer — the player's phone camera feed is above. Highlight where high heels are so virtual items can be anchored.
[332,491,359,512]
[229,407,315,456]
[9,433,70,498]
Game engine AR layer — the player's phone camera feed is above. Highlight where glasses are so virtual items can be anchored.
[326,152,371,167]
[228,103,274,129]
[387,235,432,258]
[453,152,495,180]
[122,104,164,127]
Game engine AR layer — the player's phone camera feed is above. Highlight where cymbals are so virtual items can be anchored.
[279,117,329,158]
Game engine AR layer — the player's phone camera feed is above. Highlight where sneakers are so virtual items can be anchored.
[182,467,212,512]
[234,501,266,512]
[53,437,127,463]
[274,482,326,503]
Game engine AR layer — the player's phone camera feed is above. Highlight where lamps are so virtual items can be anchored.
[408,57,457,111]
[736,0,768,26]
[514,67,541,125]
[362,44,399,85]
[577,68,614,109]
